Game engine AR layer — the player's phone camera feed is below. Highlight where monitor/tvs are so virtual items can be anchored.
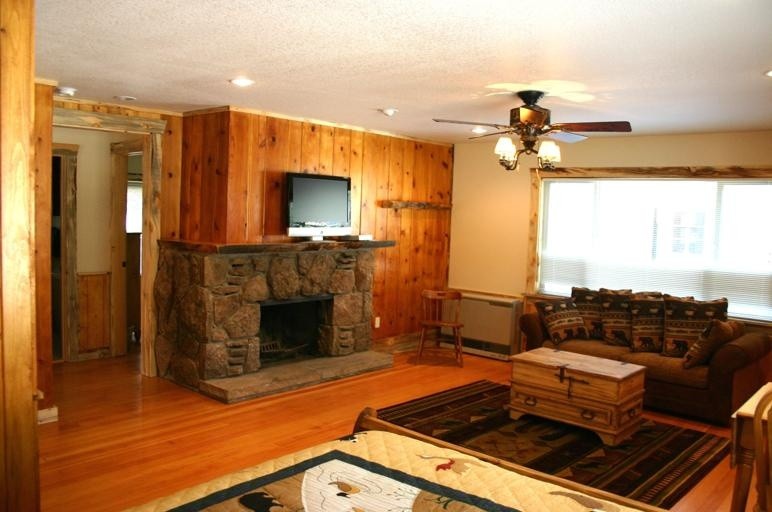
[287,172,351,243]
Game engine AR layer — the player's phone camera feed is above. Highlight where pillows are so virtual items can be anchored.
[631,291,665,352]
[599,288,632,345]
[663,294,728,358]
[682,319,743,369]
[571,287,601,337]
[535,296,589,345]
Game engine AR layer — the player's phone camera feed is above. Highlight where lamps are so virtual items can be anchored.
[494,127,562,171]
[54,87,78,98]
[378,107,399,116]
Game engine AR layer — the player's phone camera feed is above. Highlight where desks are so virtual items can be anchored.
[440,288,524,362]
[731,381,772,512]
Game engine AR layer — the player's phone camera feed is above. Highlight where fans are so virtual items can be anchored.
[432,89,633,143]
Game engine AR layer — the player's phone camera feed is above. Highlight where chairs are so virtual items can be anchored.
[415,289,464,367]
[752,390,772,512]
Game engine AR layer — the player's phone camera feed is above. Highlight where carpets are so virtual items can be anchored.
[375,377,732,511]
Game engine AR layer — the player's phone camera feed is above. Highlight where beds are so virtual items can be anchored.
[125,406,668,512]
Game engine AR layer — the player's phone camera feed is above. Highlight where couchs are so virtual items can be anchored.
[518,311,772,428]
